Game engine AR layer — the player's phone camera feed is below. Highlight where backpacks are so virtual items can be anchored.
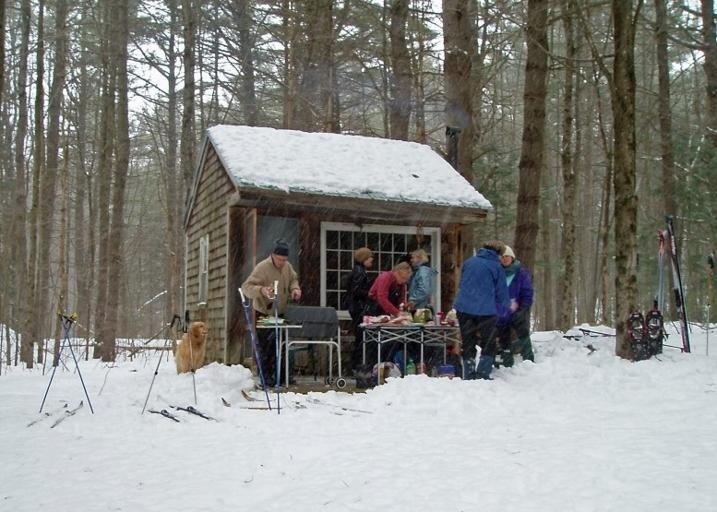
[623,309,669,361]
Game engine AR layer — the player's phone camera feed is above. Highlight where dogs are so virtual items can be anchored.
[175,320,209,375]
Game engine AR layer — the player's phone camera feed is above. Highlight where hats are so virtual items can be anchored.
[273,242,289,256]
[354,247,372,263]
[498,244,515,260]
[408,249,428,260]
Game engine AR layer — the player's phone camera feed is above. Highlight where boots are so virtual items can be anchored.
[463,335,535,380]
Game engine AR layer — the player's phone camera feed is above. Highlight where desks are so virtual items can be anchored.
[359,321,466,387]
[255,324,303,389]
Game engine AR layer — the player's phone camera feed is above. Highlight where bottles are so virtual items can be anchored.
[406,359,416,375]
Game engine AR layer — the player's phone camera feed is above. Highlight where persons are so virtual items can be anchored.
[493,244,536,368]
[239,242,303,391]
[452,239,520,381]
[342,246,375,373]
[361,260,414,369]
[398,248,440,363]
